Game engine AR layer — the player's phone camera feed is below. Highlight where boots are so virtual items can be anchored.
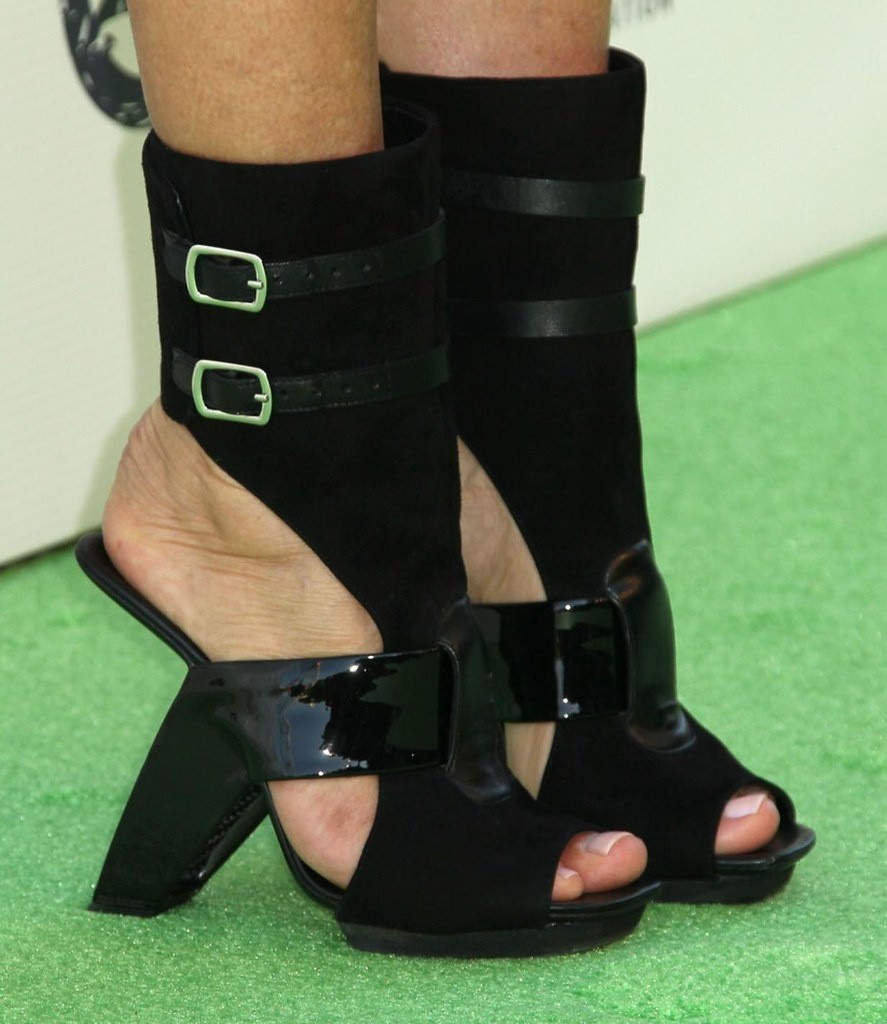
[69,124,667,959]
[376,49,818,904]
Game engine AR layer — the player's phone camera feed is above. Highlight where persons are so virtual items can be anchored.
[73,0,819,961]
